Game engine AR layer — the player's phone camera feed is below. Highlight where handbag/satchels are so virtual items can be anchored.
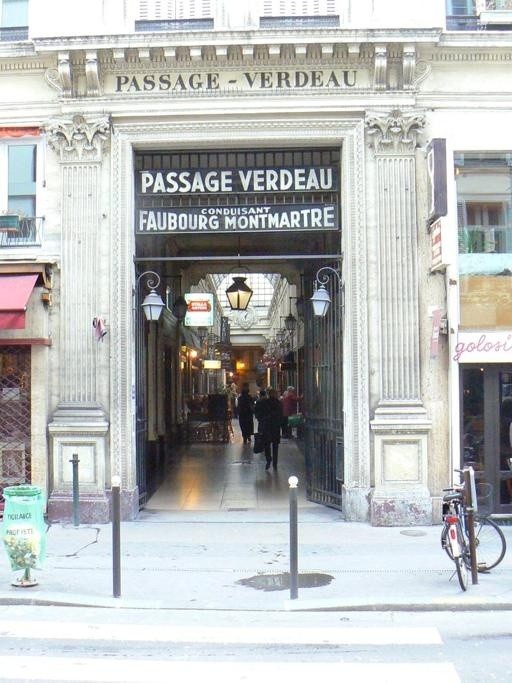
[252,431,266,453]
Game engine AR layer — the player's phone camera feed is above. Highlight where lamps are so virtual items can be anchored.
[136,235,342,356]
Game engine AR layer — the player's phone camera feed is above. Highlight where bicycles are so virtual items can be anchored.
[440,469,507,592]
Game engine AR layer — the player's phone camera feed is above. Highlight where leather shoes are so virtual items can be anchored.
[265,456,277,470]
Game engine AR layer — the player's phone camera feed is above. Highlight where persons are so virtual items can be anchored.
[233,385,304,472]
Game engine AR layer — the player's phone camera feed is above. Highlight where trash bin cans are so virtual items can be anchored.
[1,485,47,587]
